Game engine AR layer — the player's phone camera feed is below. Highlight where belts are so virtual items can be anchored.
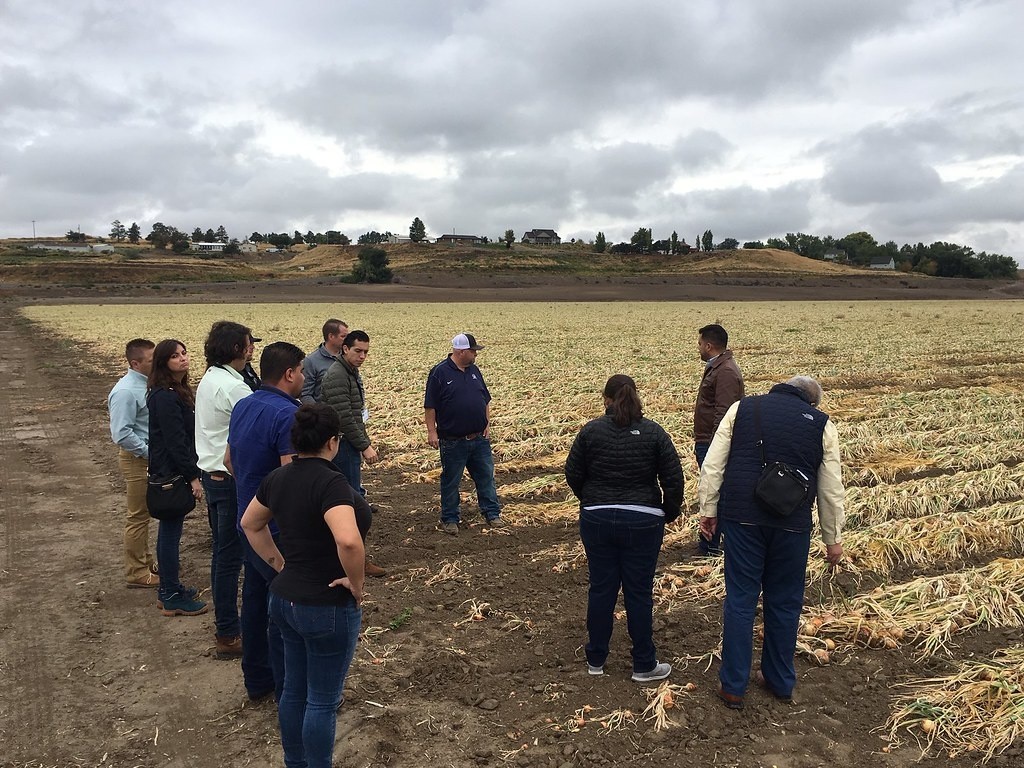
[205,470,226,477]
[443,429,484,440]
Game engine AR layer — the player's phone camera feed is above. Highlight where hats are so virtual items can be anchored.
[248,330,263,344]
[452,333,485,350]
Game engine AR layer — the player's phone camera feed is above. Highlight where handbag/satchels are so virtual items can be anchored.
[146,475,196,520]
[754,394,810,518]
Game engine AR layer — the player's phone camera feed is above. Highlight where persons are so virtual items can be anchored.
[107,336,161,590]
[424,333,505,535]
[298,318,388,578]
[145,339,210,617]
[195,322,305,705]
[697,375,844,709]
[239,397,373,768]
[681,324,746,560]
[564,374,685,681]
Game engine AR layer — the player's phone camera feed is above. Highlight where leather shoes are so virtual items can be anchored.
[779,695,792,704]
[364,561,387,577]
[717,680,744,709]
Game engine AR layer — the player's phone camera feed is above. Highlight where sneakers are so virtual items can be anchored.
[586,661,605,675]
[126,561,159,589]
[631,660,671,682]
[156,586,208,618]
[442,520,459,535]
[216,633,247,659]
[488,517,504,528]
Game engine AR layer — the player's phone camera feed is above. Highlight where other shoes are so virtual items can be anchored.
[247,683,276,704]
[337,693,345,709]
